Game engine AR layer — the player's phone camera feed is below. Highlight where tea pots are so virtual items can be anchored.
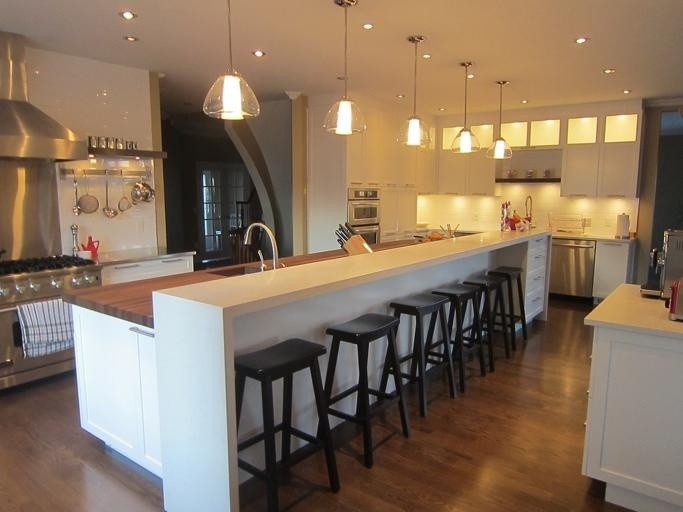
[81,235,99,257]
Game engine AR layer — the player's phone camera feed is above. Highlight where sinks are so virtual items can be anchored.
[208,265,266,278]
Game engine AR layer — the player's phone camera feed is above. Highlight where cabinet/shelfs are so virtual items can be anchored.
[71,304,163,479]
[59,147,168,181]
[101,256,195,287]
[527,234,548,323]
[380,188,418,236]
[559,112,642,198]
[416,125,495,195]
[592,239,636,299]
[496,116,561,180]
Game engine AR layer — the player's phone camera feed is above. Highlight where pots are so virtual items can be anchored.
[131,181,154,206]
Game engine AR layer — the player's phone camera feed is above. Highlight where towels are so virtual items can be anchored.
[17,298,75,360]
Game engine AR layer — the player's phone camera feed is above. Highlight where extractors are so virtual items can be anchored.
[0,32,87,164]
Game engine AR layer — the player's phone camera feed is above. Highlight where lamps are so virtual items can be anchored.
[400,34,435,146]
[203,1,261,122]
[488,79,512,160]
[322,0,367,137]
[453,60,482,155]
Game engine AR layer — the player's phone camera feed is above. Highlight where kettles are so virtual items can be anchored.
[615,212,630,240]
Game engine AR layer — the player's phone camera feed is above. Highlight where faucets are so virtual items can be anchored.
[242,221,280,271]
[525,195,533,216]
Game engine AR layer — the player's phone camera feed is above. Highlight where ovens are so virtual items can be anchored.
[0,297,73,392]
[347,187,380,244]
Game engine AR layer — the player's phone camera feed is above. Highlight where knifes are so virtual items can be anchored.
[334,221,355,248]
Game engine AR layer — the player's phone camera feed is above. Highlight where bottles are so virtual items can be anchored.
[88,135,137,150]
[500,201,512,232]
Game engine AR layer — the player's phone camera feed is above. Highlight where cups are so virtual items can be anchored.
[527,169,535,179]
[506,169,516,178]
[543,168,553,178]
[77,251,91,260]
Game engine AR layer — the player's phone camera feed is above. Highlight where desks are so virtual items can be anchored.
[582,283,683,508]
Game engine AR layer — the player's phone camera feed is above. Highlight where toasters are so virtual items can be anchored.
[667,278,683,320]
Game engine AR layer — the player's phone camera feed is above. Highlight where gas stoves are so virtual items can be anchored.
[0,254,102,298]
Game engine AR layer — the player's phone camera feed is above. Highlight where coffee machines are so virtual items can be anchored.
[639,228,683,299]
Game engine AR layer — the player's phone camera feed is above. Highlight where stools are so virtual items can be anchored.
[486,268,529,351]
[237,336,340,511]
[430,283,489,389]
[460,276,513,372]
[323,312,410,469]
[387,294,457,415]
